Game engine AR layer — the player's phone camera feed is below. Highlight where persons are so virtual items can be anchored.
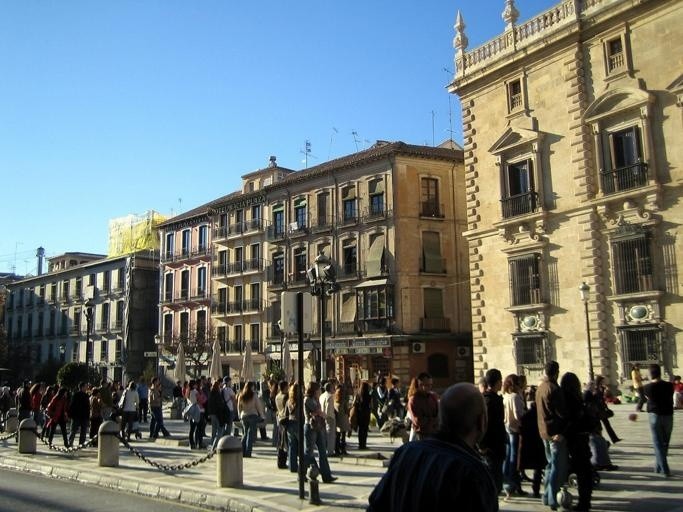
[1,360,683,511]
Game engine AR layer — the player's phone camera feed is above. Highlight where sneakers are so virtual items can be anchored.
[323,475,338,483]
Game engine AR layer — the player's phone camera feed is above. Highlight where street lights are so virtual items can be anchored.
[579,281,596,388]
[305,250,338,375]
[57,343,66,363]
[81,296,95,386]
[153,333,160,379]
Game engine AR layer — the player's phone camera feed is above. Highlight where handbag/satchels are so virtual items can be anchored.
[45,407,54,419]
[116,388,128,416]
[309,415,326,431]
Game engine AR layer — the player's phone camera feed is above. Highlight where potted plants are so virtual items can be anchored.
[456,345,472,357]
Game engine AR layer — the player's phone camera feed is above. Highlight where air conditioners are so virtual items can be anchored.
[412,342,425,353]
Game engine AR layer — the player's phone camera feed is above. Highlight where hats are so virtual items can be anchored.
[222,375,231,385]
